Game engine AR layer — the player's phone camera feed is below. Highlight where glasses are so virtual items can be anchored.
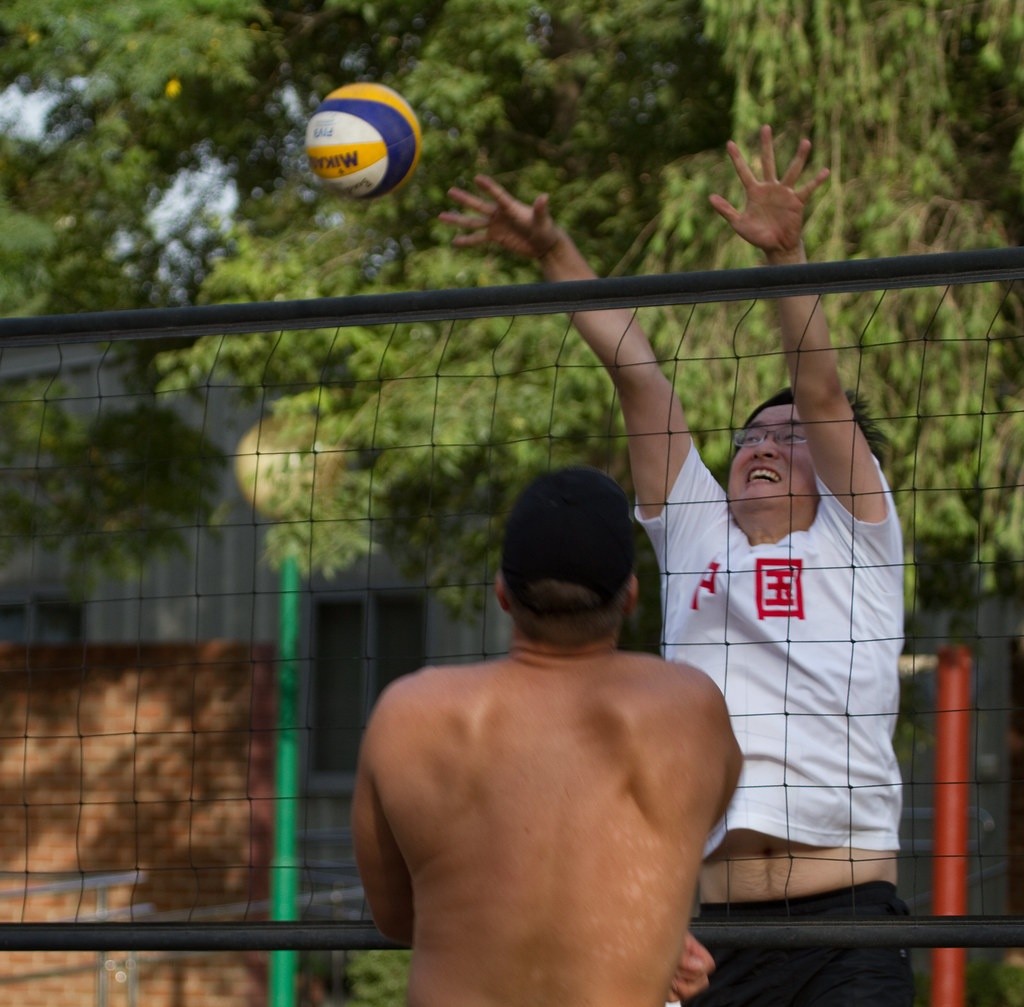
[732,425,808,447]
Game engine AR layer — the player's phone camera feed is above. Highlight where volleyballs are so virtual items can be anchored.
[302,81,422,202]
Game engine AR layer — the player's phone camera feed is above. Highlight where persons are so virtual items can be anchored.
[437,126,918,1007]
[348,466,748,1007]
